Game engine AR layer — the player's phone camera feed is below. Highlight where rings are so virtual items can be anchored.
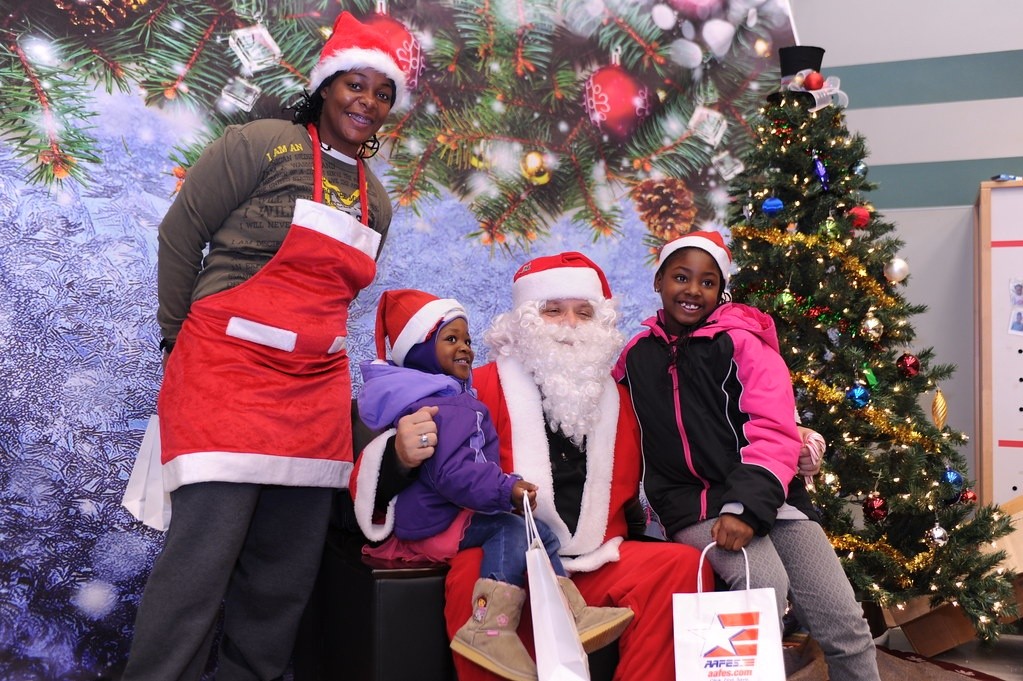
[420,434,429,448]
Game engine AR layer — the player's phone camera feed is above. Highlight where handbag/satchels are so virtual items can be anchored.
[522,490,591,681]
[119,414,172,532]
[672,540,786,681]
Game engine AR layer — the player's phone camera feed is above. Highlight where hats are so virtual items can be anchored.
[653,230,732,289]
[513,252,613,310]
[309,11,427,114]
[375,290,469,367]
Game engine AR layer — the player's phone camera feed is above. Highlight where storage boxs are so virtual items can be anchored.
[880,495,1023,658]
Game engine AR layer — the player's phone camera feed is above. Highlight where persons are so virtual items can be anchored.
[611,230,885,681]
[356,289,635,681]
[121,10,421,679]
[346,251,826,681]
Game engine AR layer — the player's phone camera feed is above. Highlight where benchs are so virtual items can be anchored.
[296,561,456,681]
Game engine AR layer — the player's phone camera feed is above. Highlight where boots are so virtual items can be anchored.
[450,578,538,681]
[555,575,634,654]
